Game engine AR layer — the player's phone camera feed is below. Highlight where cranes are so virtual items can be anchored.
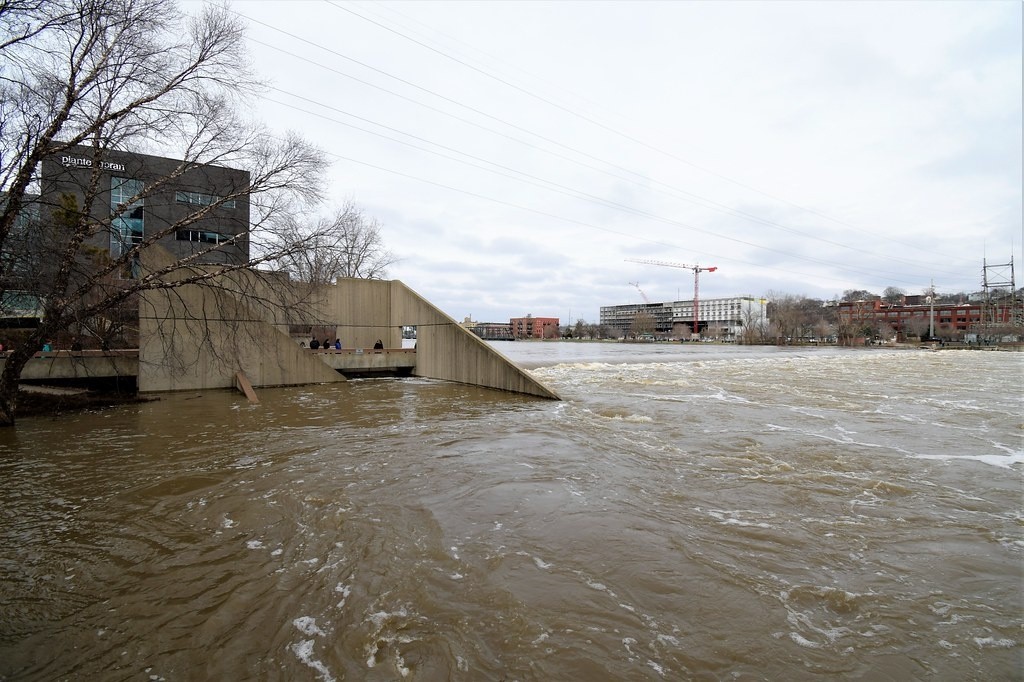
[623,257,718,335]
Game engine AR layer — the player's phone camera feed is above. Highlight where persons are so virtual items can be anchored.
[310,337,320,354]
[323,339,331,354]
[300,342,306,347]
[374,338,383,349]
[43,340,53,358]
[71,337,81,358]
[102,340,110,351]
[335,339,341,354]
[0,338,8,359]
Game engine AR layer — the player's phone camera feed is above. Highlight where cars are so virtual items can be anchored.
[809,338,821,343]
[925,336,943,343]
[724,337,736,343]
[701,338,713,343]
